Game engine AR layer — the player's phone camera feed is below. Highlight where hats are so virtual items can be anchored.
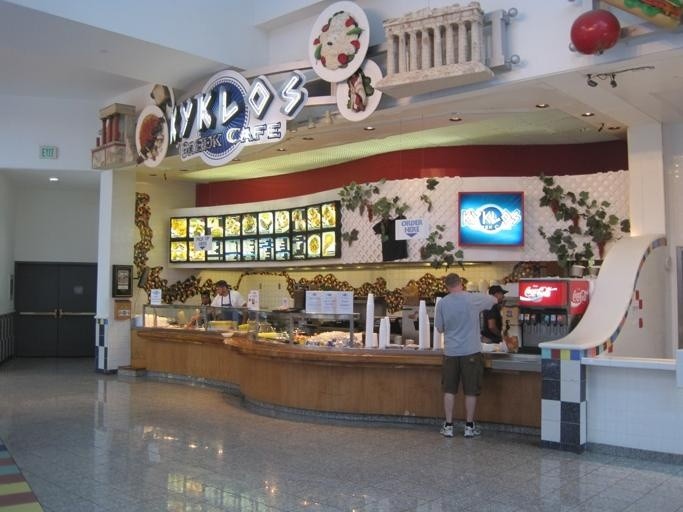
[489,285,509,296]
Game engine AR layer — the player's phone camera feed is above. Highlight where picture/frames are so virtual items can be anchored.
[112,263,133,297]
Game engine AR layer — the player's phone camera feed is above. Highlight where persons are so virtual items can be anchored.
[434,273,499,437]
[198,289,212,328]
[483,285,509,343]
[190,279,248,325]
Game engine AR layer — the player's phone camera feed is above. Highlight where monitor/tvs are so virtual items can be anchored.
[457,190,526,248]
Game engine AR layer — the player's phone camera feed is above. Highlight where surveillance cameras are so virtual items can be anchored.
[610,79,617,88]
[587,79,597,87]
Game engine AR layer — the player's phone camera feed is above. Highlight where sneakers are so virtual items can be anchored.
[439,421,454,437]
[463,421,483,437]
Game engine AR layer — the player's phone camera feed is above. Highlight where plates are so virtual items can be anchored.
[134,105,169,169]
[335,58,383,123]
[307,1,370,84]
[170,210,335,261]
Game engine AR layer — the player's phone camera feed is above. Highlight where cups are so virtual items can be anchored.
[363,292,443,350]
